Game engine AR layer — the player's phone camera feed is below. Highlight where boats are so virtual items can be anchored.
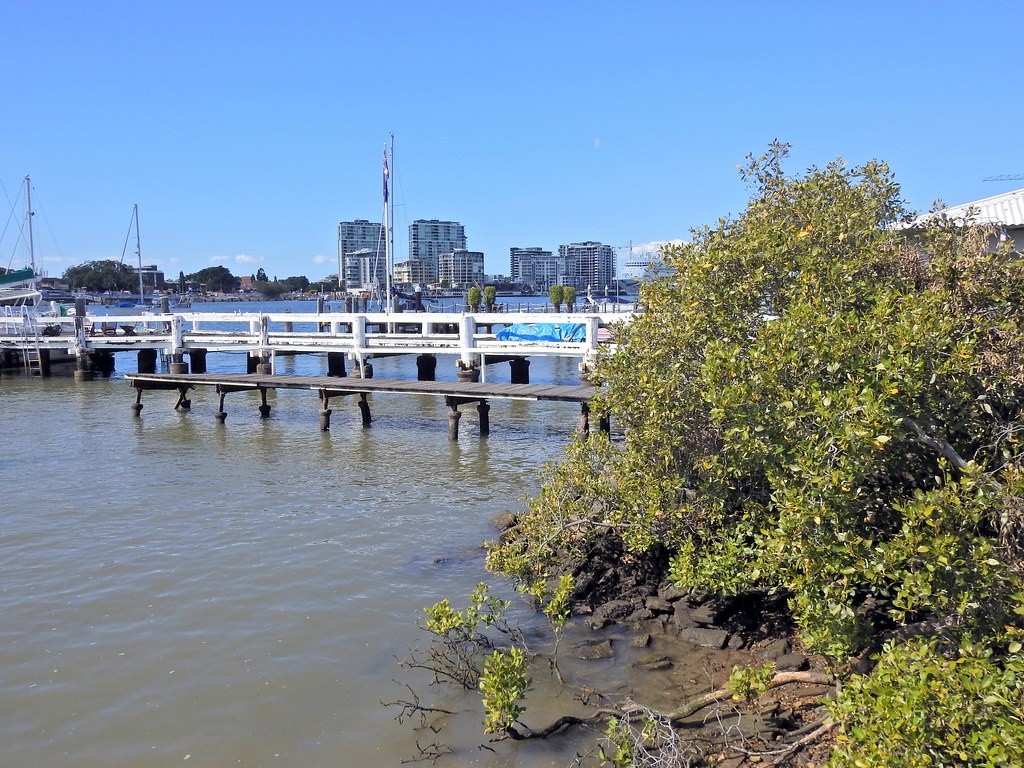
[0,172,193,313]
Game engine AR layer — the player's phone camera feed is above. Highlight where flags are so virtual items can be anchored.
[382,154,389,203]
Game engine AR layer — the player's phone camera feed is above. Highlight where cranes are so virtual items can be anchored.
[617,239,649,261]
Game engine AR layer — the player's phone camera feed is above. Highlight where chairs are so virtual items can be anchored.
[100,322,118,336]
[120,314,139,336]
[85,322,94,337]
